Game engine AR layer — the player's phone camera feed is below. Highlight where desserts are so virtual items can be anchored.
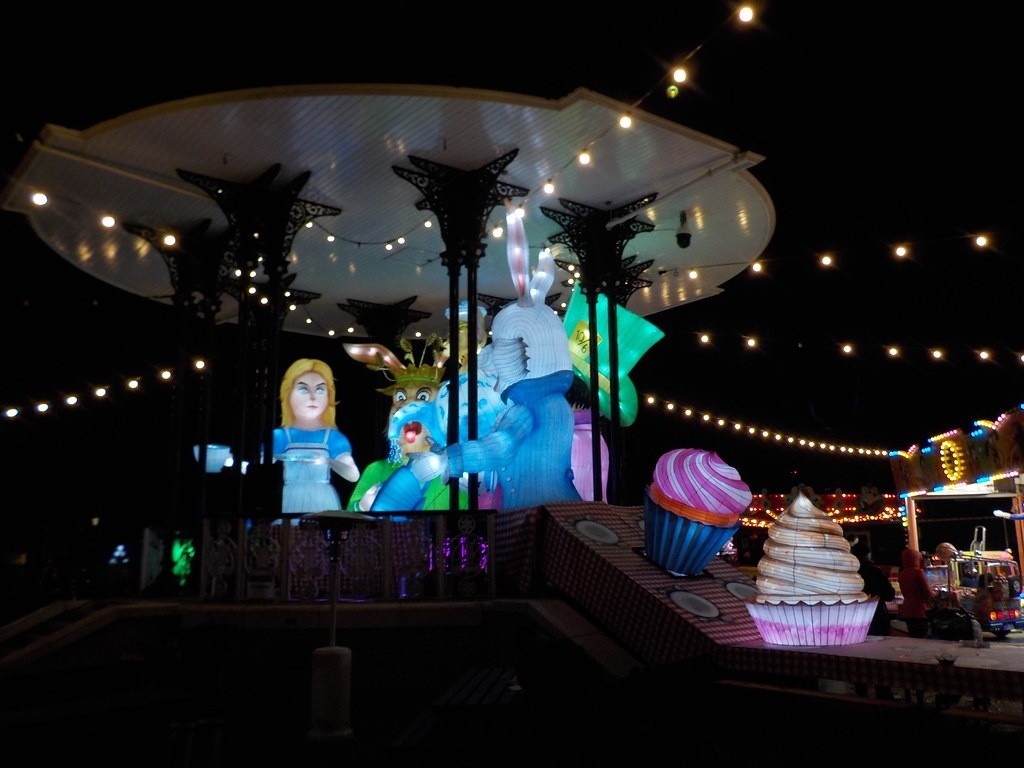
[745,491,879,645]
[644,449,752,571]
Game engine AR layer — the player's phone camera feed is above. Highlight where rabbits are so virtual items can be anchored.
[404,199,585,510]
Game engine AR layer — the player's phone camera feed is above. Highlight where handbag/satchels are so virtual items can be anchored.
[925,592,974,640]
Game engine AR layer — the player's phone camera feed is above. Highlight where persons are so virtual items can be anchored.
[850,542,895,636]
[723,549,752,566]
[897,549,939,638]
[960,565,979,588]
